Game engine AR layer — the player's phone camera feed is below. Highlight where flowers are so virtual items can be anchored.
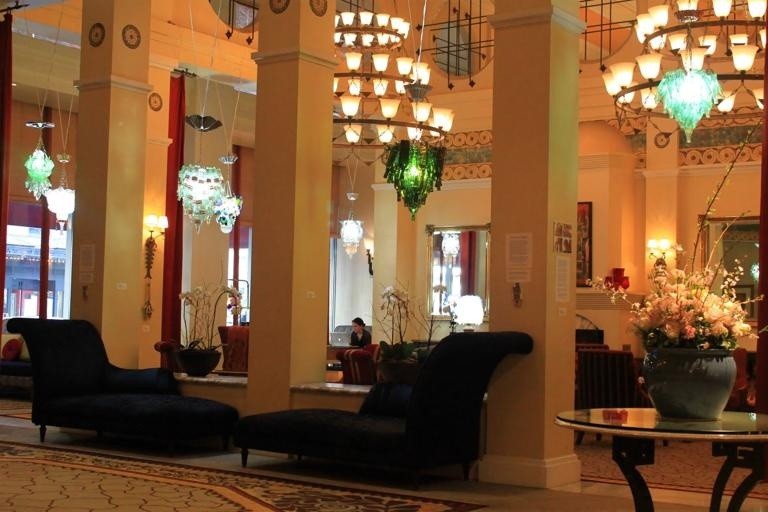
[376,279,418,372]
[592,115,763,352]
[175,259,240,351]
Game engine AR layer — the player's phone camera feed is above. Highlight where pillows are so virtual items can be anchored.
[20,340,31,360]
[359,356,422,421]
[87,363,177,393]
[1,334,22,360]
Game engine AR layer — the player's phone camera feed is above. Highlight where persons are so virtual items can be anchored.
[349,317,371,346]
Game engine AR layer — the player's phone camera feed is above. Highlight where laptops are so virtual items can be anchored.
[330,332,351,347]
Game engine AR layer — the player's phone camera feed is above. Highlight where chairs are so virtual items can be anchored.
[576,329,603,344]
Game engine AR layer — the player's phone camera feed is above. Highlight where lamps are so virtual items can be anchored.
[340,146,364,261]
[145,213,169,238]
[441,230,460,265]
[178,1,223,236]
[601,0,766,148]
[334,1,455,145]
[383,0,447,220]
[649,238,670,279]
[13,0,65,201]
[453,295,485,333]
[212,33,248,235]
[44,86,75,234]
[657,11,723,146]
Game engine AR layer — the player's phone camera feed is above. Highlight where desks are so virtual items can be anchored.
[556,408,768,512]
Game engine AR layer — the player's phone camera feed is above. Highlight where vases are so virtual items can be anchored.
[177,349,223,376]
[643,347,736,419]
[379,353,416,386]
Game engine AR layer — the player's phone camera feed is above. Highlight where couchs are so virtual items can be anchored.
[335,350,372,385]
[576,344,608,352]
[371,343,381,361]
[0,318,32,401]
[217,326,249,373]
[6,318,239,456]
[574,350,637,446]
[235,331,533,490]
[335,324,372,346]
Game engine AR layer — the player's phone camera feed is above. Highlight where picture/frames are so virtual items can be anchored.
[577,202,593,288]
[726,283,753,317]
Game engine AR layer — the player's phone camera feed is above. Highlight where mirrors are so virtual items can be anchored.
[700,215,759,326]
[423,222,492,323]
